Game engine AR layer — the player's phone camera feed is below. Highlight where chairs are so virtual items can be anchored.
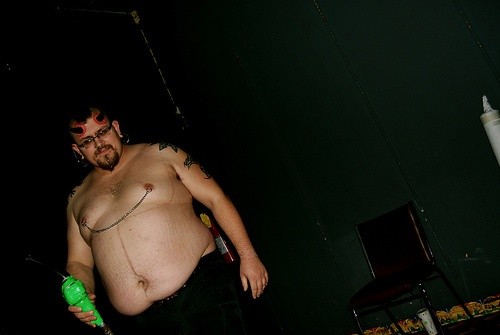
[348,200,475,335]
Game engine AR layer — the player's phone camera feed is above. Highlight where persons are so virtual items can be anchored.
[64,98,271,334]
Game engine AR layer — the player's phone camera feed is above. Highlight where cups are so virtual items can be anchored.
[416,307,438,335]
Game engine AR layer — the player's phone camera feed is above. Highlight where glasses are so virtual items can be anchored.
[76,125,113,149]
